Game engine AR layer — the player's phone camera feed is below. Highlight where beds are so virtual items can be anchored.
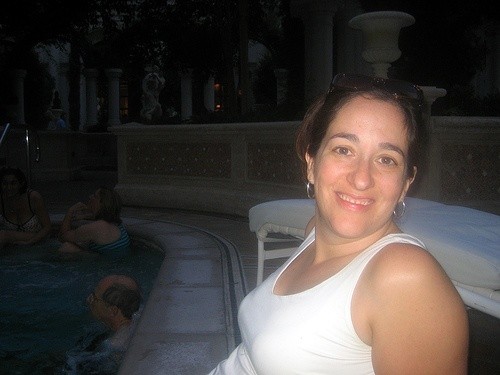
[247,196,500,318]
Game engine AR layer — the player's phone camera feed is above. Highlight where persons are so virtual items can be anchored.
[0,181,145,353]
[205,69,468,375]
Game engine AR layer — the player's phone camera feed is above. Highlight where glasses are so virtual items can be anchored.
[328,72,423,110]
